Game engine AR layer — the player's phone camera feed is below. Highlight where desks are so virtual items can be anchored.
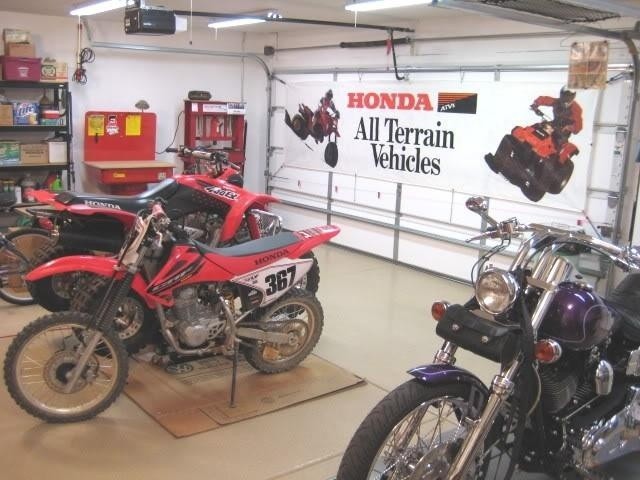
[83,159,175,195]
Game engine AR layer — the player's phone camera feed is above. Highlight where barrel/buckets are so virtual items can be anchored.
[21,173,36,201]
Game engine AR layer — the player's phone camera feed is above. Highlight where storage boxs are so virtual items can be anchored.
[0,25,42,81]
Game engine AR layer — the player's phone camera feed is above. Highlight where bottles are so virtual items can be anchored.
[0,175,63,204]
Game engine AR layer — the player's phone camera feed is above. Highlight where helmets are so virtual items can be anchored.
[559,86,576,103]
[326,89,333,97]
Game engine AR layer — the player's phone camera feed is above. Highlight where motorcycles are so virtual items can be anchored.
[336,197,640,479]
[284,105,339,168]
[484,103,579,202]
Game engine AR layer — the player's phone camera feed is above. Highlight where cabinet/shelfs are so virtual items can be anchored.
[0,81,70,194]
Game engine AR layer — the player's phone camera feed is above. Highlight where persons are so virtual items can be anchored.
[529,84,583,165]
[312,89,341,136]
